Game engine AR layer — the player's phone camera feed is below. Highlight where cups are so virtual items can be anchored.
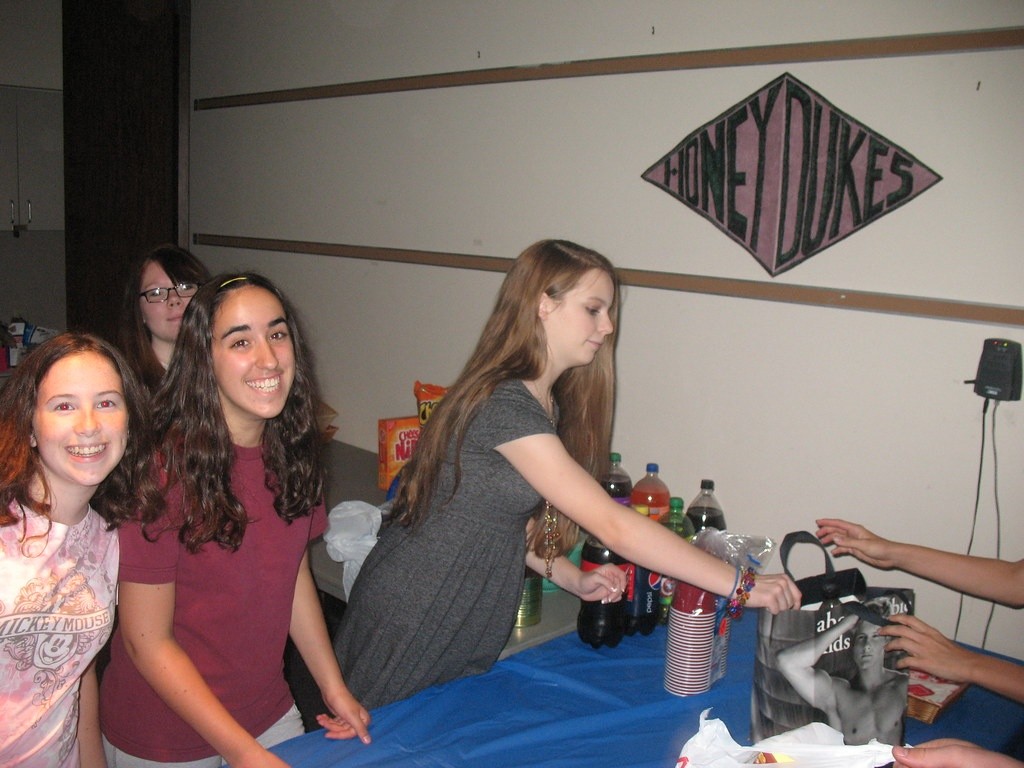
[663,580,732,698]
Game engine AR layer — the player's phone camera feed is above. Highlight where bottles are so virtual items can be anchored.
[577,453,723,649]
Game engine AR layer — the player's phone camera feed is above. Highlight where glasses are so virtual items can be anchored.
[139,282,199,304]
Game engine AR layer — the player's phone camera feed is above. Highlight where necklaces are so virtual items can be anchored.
[530,380,561,580]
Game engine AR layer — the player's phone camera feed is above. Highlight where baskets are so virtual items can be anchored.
[906,678,968,724]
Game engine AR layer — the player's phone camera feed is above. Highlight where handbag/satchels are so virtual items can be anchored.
[751,532,915,748]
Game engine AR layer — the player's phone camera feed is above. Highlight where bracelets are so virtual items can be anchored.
[726,567,757,619]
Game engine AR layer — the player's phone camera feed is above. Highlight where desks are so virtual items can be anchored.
[311,438,580,661]
[223,610,1024,768]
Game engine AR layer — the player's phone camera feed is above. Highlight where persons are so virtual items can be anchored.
[0,335,168,768]
[101,271,372,768]
[90,245,215,688]
[814,518,1024,705]
[892,737,1024,768]
[777,600,910,747]
[332,240,803,711]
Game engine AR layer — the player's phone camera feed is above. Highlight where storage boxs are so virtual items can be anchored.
[378,416,422,490]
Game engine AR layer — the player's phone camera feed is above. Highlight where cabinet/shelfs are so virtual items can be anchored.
[0,85,65,231]
